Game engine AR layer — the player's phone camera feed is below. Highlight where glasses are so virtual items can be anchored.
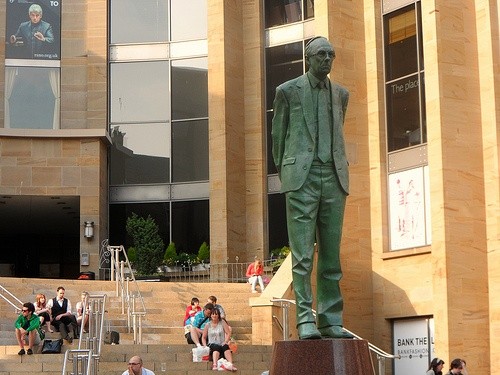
[128,363,138,365]
[22,309,28,312]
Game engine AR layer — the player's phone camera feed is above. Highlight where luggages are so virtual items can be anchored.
[104,311,120,345]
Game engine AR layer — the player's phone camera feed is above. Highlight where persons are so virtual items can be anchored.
[425,357,445,375]
[76,290,95,333]
[444,358,468,375]
[33,293,54,333]
[46,287,80,343]
[272,36,355,339]
[246,258,265,294]
[202,309,238,371]
[184,295,227,348]
[121,355,155,375]
[10,4,55,59]
[14,302,45,355]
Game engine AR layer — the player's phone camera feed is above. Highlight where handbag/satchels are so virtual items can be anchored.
[184,332,194,344]
[263,276,270,284]
[192,343,210,363]
[41,338,63,353]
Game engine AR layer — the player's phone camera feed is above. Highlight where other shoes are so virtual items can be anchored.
[27,349,33,353]
[262,289,264,293]
[83,329,86,333]
[232,364,237,371]
[18,349,25,354]
[48,330,54,332]
[75,335,80,339]
[252,290,258,293]
[67,336,73,343]
[212,364,217,371]
